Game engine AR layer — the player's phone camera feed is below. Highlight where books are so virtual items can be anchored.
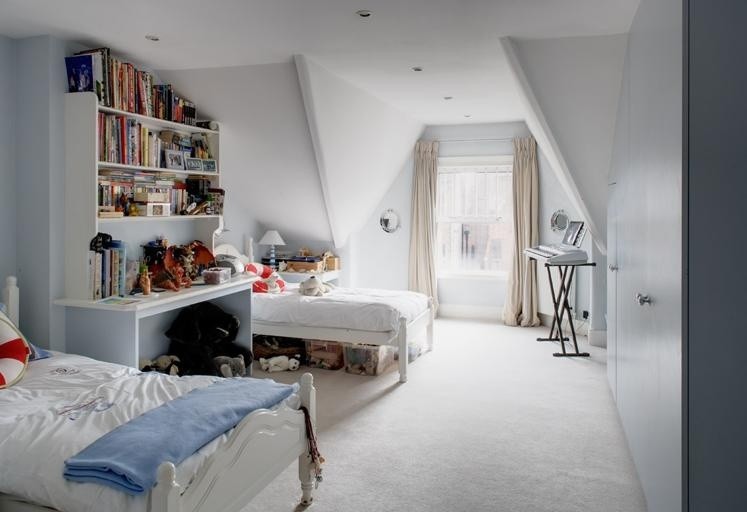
[65,48,227,219]
[89,235,126,299]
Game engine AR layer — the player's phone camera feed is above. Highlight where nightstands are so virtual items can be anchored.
[278,268,341,289]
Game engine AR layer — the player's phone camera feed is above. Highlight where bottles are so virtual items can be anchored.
[141,266,150,295]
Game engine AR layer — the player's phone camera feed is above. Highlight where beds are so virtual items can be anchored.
[213,235,437,385]
[1,273,324,512]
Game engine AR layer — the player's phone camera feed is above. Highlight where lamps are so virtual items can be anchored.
[259,228,288,267]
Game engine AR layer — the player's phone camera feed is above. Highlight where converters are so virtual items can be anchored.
[583,310,588,319]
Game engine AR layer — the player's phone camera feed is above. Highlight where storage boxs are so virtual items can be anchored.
[300,338,398,378]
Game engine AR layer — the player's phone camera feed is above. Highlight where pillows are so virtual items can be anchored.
[1,304,53,397]
[215,252,288,293]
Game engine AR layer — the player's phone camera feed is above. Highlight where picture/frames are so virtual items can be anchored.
[164,148,219,173]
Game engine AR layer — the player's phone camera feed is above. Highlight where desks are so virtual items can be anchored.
[57,273,263,375]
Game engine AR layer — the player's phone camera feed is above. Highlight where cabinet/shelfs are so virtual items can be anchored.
[64,90,226,225]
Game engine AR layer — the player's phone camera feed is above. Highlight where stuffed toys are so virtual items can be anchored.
[139,303,303,380]
[126,237,215,295]
[299,276,336,296]
